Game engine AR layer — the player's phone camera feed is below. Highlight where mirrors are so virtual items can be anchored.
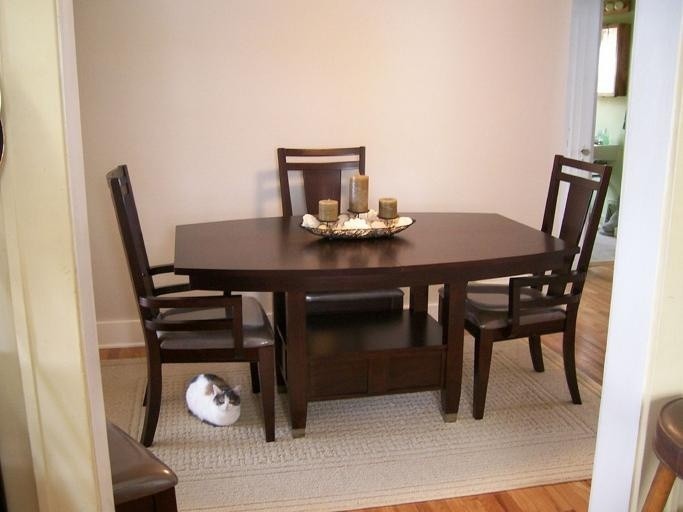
[596,22,631,98]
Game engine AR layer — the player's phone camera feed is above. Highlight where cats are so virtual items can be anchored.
[183,372,244,428]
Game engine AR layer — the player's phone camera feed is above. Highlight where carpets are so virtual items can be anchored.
[93,326,603,511]
